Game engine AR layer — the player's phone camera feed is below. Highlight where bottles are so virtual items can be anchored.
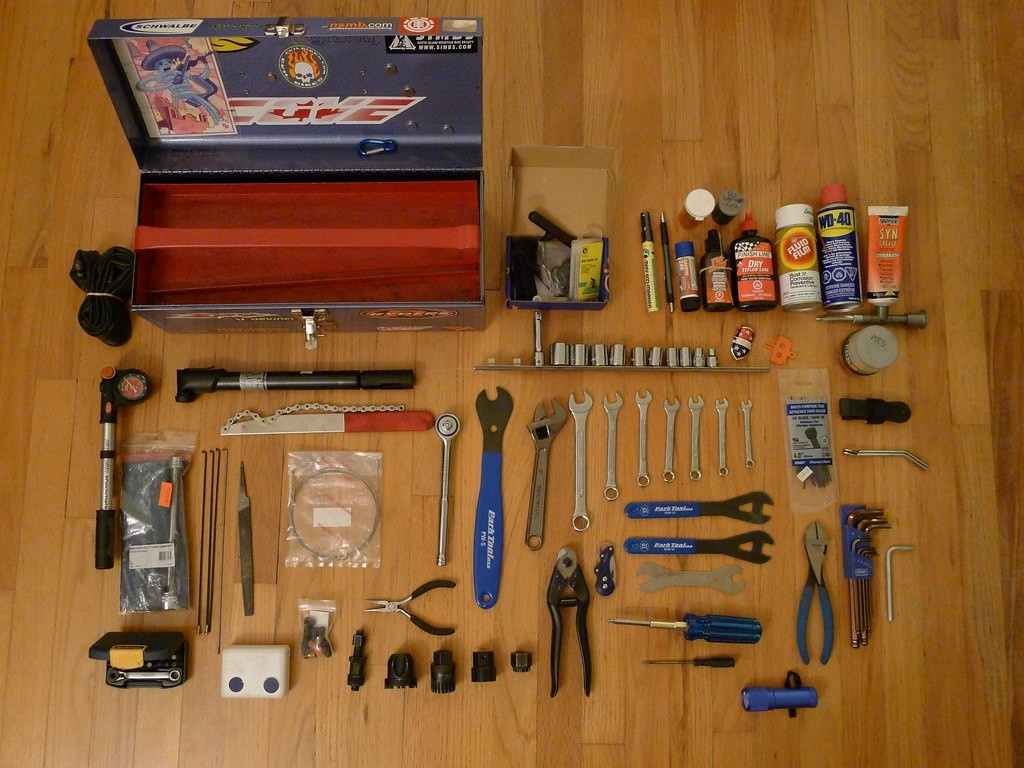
[711,189,746,226]
[678,189,716,232]
[775,203,823,313]
[699,229,733,312]
[837,325,899,376]
[729,211,781,313]
[815,183,864,313]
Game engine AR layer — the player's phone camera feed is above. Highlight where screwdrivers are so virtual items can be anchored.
[605,613,763,644]
[640,657,735,668]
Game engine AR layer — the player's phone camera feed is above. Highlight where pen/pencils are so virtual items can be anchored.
[660,211,677,313]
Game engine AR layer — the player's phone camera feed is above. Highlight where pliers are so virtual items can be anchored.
[545,546,593,699]
[363,579,457,636]
[796,519,835,666]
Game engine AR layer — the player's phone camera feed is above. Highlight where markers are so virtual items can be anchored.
[640,211,659,312]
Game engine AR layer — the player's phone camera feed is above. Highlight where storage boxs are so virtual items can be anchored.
[86,17,486,349]
[504,145,615,311]
